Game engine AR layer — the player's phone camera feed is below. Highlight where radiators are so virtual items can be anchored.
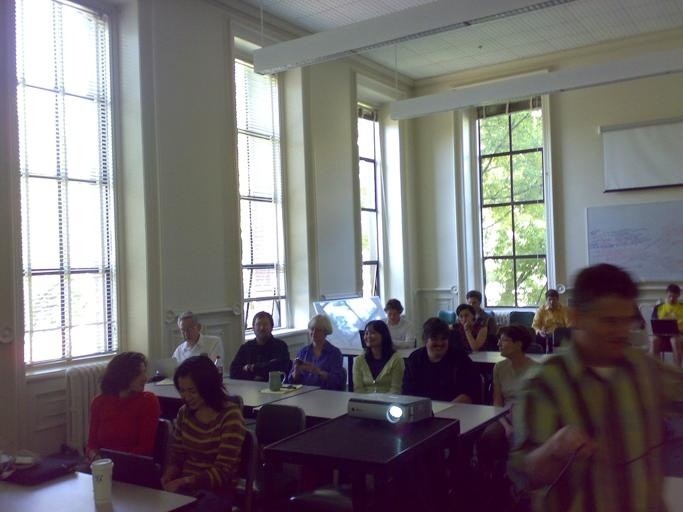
[68,361,119,458]
[273,333,312,364]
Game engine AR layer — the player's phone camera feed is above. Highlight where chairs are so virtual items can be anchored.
[437,310,457,327]
[545,325,572,352]
[447,371,497,493]
[508,310,538,329]
[525,327,545,354]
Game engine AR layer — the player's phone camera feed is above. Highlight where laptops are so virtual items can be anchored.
[0,457,78,485]
[650,320,679,337]
[97,447,203,497]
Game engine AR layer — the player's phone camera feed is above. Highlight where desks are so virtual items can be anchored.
[0,448,197,511]
[262,411,461,512]
[252,385,509,510]
[404,346,562,399]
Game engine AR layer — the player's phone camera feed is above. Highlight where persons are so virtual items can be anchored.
[229,311,290,383]
[287,314,346,392]
[509,265,683,512]
[383,298,415,349]
[465,290,496,339]
[648,284,682,367]
[81,352,161,473]
[172,310,224,366]
[352,320,404,396]
[492,324,542,443]
[403,317,482,404]
[530,290,573,339]
[447,304,487,353]
[159,354,246,512]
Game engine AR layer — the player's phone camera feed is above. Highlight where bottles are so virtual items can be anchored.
[214,356,223,382]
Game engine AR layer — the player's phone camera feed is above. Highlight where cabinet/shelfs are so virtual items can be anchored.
[143,372,322,417]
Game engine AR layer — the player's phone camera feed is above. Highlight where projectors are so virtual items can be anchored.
[348,391,432,429]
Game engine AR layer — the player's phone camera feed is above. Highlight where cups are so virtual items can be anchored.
[89,458,114,505]
[268,371,286,392]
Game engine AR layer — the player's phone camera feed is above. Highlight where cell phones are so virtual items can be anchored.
[295,357,303,366]
